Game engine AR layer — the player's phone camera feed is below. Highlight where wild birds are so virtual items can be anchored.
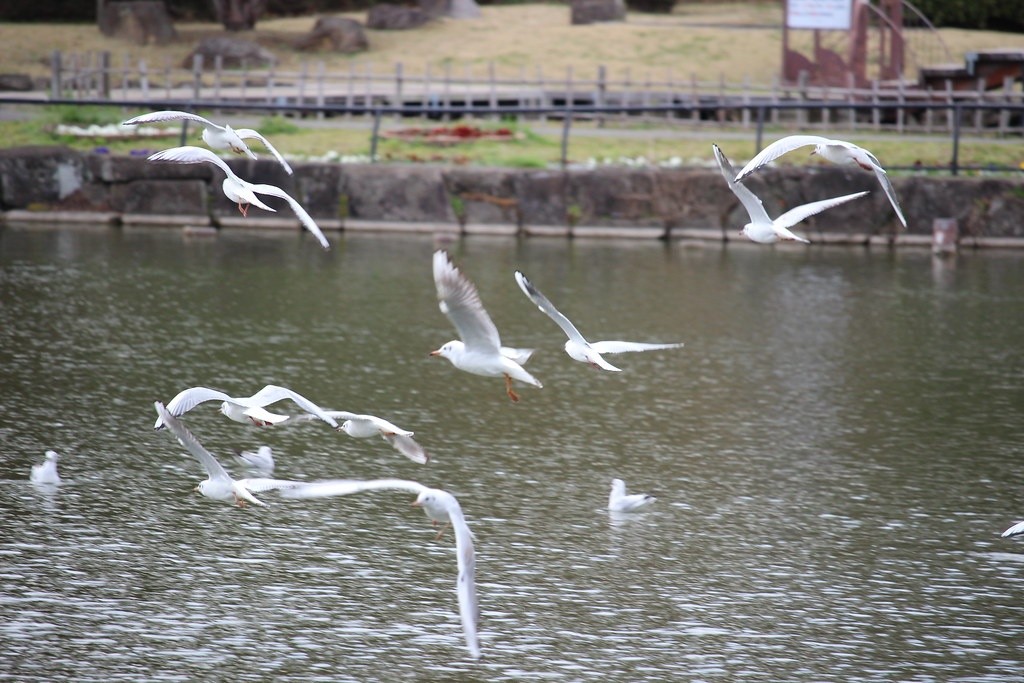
[145,145,330,248]
[153,384,339,432]
[608,478,658,512]
[734,134,908,229]
[512,268,684,372]
[153,401,305,508]
[280,477,482,659]
[1001,520,1024,547]
[29,450,65,487]
[123,111,294,175]
[429,248,544,403]
[295,411,429,466]
[231,446,276,470]
[711,142,868,242]
